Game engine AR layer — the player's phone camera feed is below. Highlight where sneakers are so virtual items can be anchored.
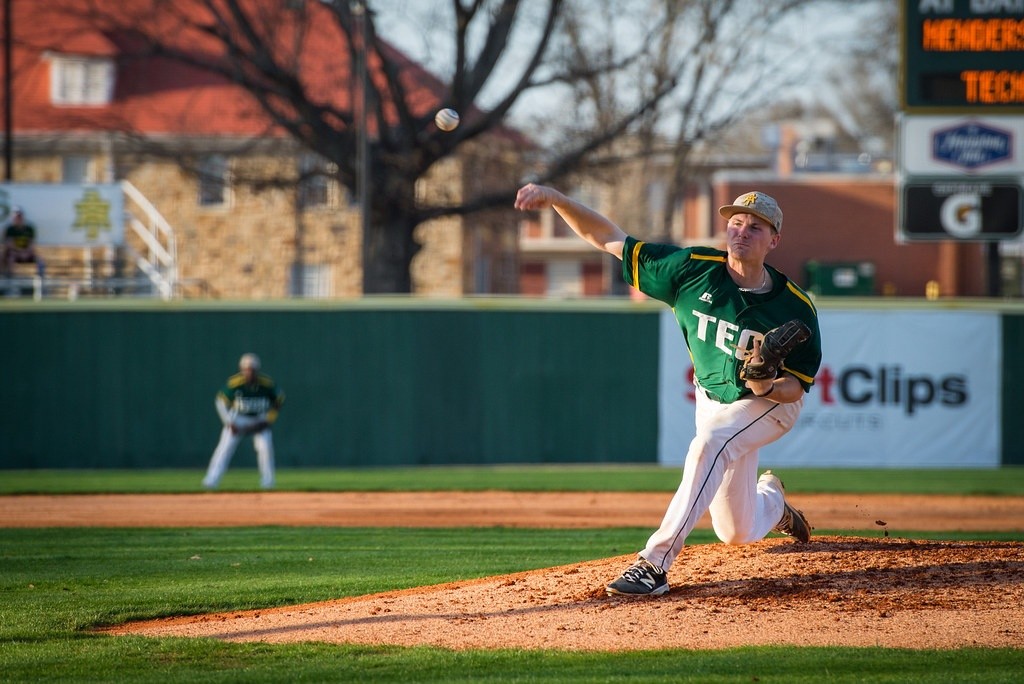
[758,473,811,543]
[605,559,670,597]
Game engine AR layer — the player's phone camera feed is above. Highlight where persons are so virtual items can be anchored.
[514,183,822,599]
[0,207,46,277]
[203,353,284,487]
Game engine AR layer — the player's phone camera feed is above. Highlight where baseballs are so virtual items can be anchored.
[434,108,460,131]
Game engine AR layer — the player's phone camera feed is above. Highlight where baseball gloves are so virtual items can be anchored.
[739,319,812,381]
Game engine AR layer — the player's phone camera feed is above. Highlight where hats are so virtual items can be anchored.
[718,190,784,234]
